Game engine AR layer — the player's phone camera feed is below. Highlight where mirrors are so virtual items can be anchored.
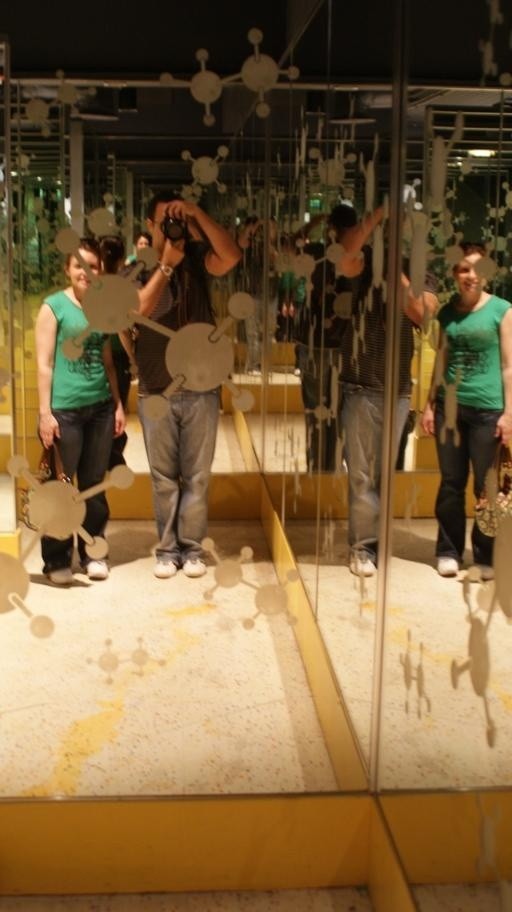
[3,2,511,894]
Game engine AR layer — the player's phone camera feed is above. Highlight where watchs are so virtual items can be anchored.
[159,264,173,277]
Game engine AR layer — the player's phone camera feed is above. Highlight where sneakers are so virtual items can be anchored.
[468,564,496,583]
[437,557,460,578]
[181,556,208,578]
[47,567,74,584]
[348,550,377,578]
[153,559,178,578]
[86,559,109,580]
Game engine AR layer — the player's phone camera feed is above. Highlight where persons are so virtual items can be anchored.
[34,237,126,586]
[99,235,137,472]
[116,191,243,578]
[124,232,152,268]
[238,199,512,584]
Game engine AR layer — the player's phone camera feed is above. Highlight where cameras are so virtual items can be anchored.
[161,208,188,242]
